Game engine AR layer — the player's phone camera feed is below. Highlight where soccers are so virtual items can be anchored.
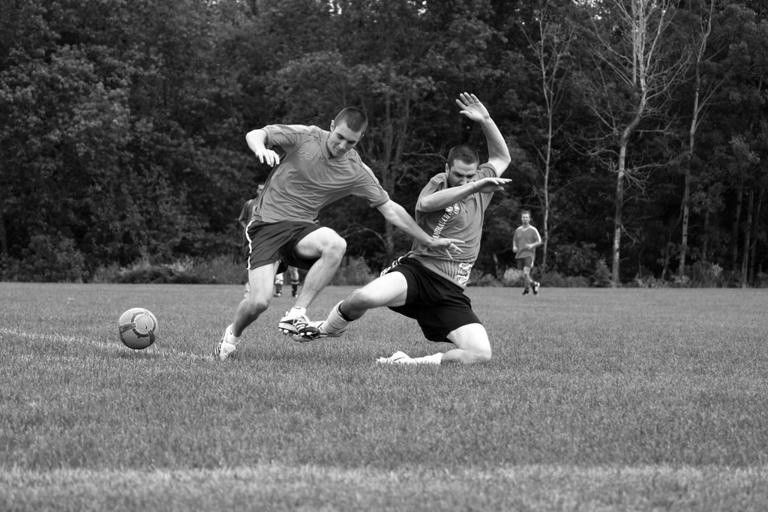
[117,307,158,348]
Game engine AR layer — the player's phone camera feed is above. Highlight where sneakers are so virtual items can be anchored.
[375,349,415,366]
[522,282,540,295]
[213,325,241,361]
[278,313,344,341]
[273,291,299,298]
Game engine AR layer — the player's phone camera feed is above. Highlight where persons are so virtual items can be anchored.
[308,93,511,364]
[215,106,466,362]
[511,210,542,296]
[274,256,299,297]
[238,181,266,297]
